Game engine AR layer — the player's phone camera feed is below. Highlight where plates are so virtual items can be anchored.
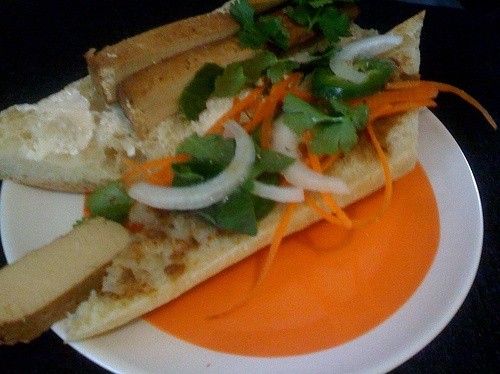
[0,77,484,374]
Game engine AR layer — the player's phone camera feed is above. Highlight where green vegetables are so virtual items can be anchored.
[0,0,500,344]
